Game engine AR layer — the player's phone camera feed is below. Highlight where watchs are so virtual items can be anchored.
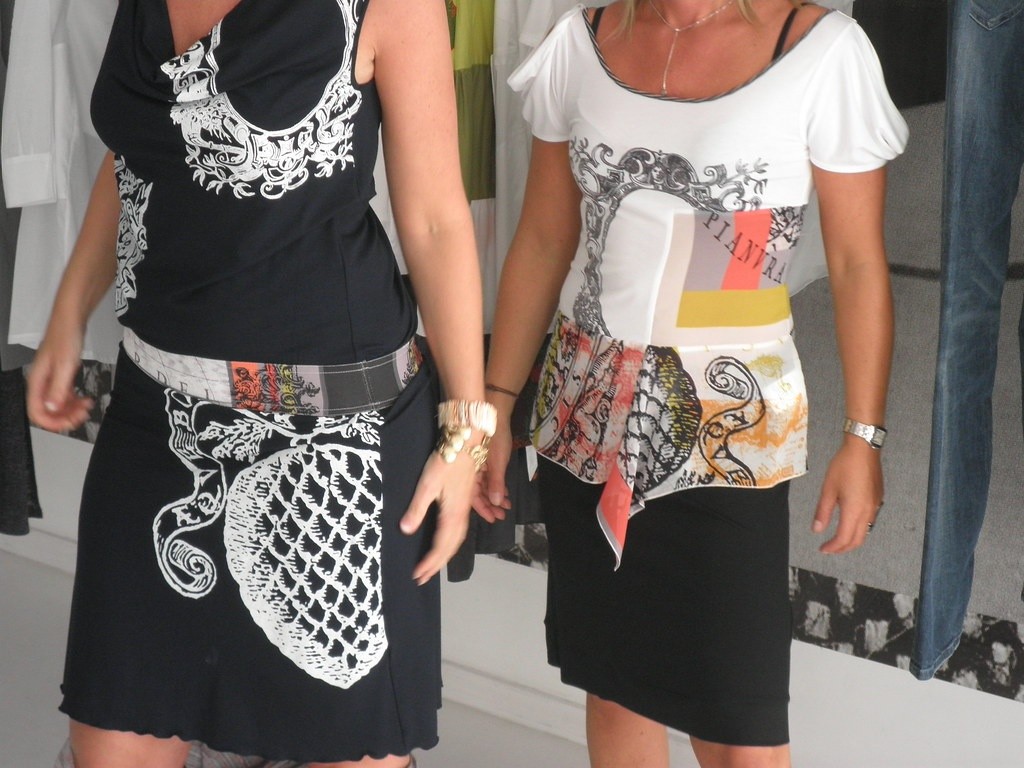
[843,416,887,450]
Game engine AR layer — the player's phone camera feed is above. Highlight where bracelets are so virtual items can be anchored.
[485,383,520,400]
[435,400,499,473]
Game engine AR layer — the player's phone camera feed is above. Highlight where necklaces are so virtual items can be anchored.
[648,0,732,96]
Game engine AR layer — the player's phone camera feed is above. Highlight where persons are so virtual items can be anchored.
[26,1,482,767]
[472,0,913,767]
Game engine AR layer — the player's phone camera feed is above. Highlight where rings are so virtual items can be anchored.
[866,522,874,535]
